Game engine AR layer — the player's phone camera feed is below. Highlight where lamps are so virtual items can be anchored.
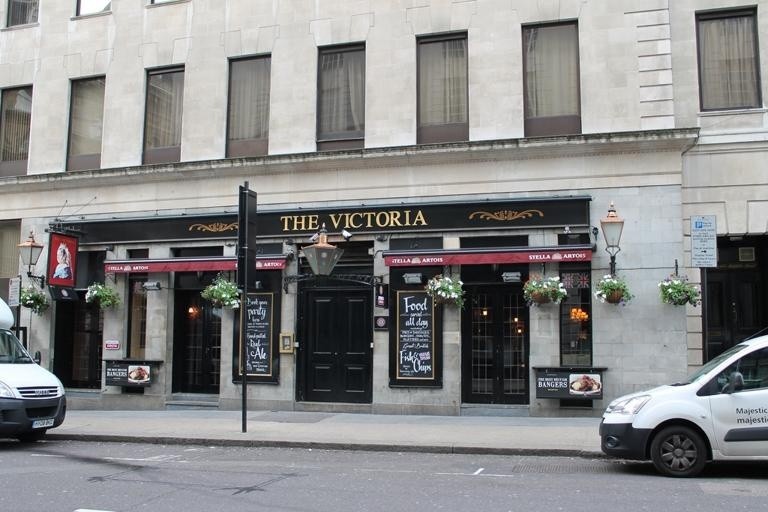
[300,224,344,278]
[16,230,45,290]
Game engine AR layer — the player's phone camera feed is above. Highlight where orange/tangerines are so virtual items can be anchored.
[571,308,589,322]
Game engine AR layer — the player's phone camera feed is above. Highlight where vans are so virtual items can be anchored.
[1,297,67,443]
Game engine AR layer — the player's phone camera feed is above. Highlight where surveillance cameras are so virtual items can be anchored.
[308,232,319,242]
[342,230,353,240]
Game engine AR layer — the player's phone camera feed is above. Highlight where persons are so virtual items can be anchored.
[53,243,72,280]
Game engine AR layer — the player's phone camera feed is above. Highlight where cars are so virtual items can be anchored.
[599,332,767,478]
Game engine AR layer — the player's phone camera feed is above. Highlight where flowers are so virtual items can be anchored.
[523,272,568,306]
[594,274,634,306]
[424,274,467,310]
[84,282,119,310]
[19,287,48,314]
[201,277,242,310]
[657,271,700,308]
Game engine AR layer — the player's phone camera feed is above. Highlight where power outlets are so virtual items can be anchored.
[601,201,625,279]
